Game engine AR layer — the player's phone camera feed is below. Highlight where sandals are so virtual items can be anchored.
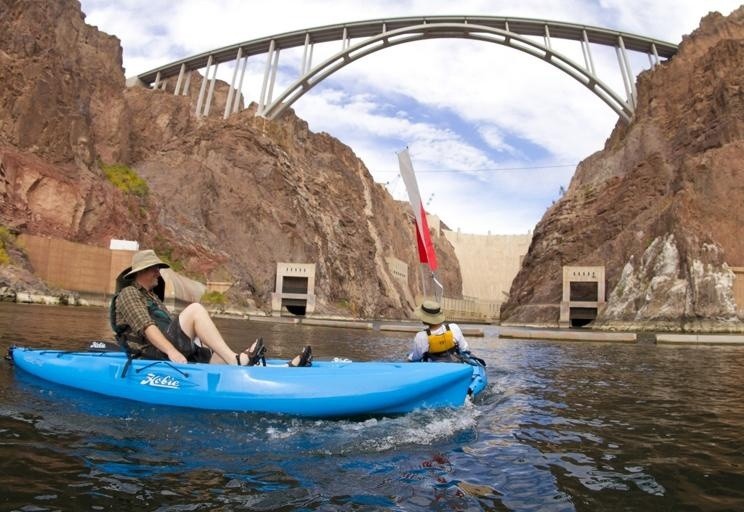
[236,337,263,366]
[286,346,311,367]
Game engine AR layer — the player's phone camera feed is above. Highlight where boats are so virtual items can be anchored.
[394,348,488,401]
[5,343,473,423]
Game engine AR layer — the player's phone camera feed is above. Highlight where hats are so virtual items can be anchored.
[414,300,445,326]
[123,250,170,278]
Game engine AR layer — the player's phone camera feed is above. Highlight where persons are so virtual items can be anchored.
[404,301,469,362]
[110,250,312,366]
